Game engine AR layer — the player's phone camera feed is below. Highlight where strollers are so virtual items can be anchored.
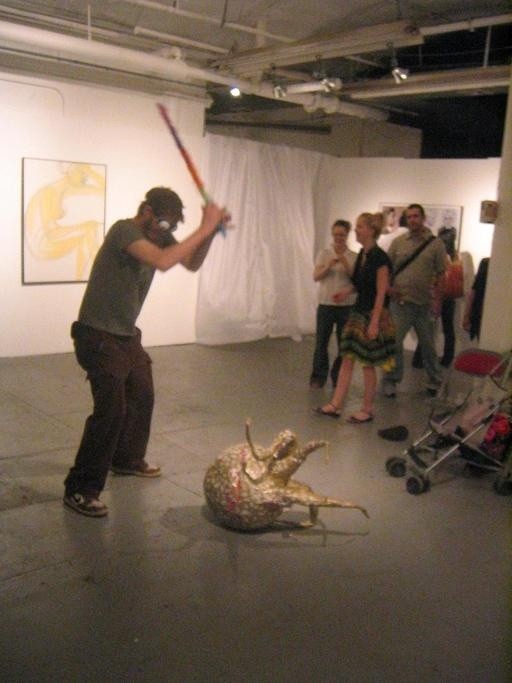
[385,347,511,497]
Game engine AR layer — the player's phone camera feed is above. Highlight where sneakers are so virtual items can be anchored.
[62,491,110,517]
[424,382,439,396]
[382,379,397,399]
[411,359,424,370]
[439,355,454,369]
[110,460,164,479]
[308,379,321,392]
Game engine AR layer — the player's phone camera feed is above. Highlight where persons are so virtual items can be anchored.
[311,211,393,424]
[383,203,446,400]
[378,208,409,253]
[411,226,460,369]
[61,182,237,517]
[462,256,490,342]
[308,217,361,390]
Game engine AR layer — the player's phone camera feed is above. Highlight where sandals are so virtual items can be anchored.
[312,402,342,419]
[345,409,376,424]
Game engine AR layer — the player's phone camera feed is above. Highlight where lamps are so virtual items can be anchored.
[268,42,411,98]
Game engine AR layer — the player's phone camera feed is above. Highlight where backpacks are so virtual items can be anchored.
[441,252,467,300]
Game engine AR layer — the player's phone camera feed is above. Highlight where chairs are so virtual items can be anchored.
[421,351,507,432]
